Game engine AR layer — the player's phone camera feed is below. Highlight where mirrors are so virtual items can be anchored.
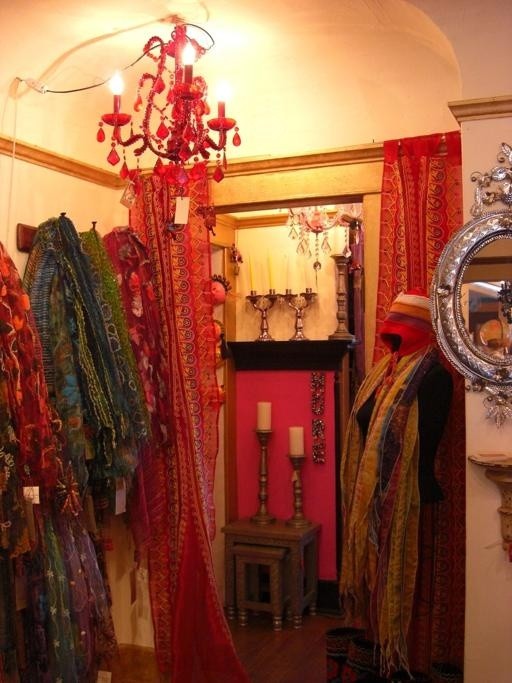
[429,142,512,430]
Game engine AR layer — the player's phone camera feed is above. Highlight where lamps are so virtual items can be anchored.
[287,205,363,270]
[97,26,242,190]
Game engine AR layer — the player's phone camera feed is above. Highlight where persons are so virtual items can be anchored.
[340,284,457,521]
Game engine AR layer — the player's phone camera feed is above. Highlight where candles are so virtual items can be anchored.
[249,253,314,293]
[255,401,305,455]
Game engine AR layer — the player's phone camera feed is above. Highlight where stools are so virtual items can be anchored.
[225,518,322,631]
